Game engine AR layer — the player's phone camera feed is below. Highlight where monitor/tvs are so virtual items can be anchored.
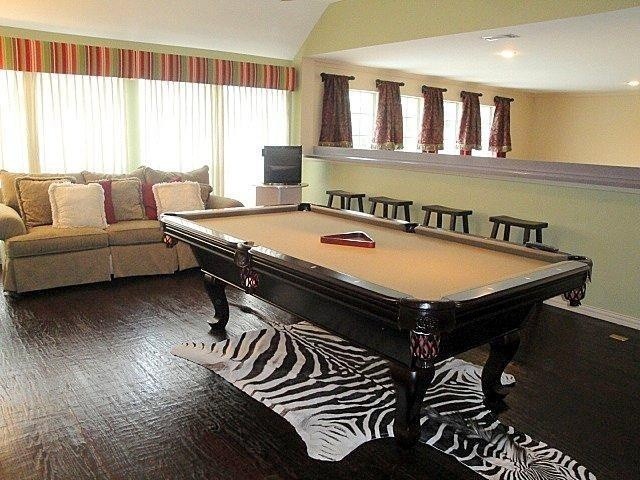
[264,146,302,185]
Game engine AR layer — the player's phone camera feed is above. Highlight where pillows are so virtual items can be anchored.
[0,165,214,228]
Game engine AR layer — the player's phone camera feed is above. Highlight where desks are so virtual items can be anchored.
[253,182,309,206]
[158,203,594,480]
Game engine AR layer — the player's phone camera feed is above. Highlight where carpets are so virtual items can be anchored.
[169,318,598,480]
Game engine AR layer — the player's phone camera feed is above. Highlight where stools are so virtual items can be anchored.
[368,197,413,222]
[489,215,548,312]
[326,189,365,213]
[421,205,472,233]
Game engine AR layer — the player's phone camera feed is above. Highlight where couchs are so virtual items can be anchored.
[0,188,245,298]
